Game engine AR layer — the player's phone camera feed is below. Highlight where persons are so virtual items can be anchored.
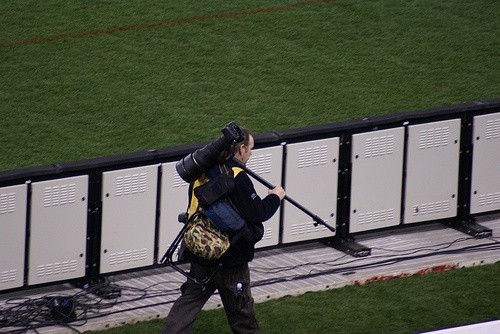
[157,126,287,334]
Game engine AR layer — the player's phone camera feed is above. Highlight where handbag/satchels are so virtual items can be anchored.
[194,172,236,207]
[183,212,230,261]
[203,199,246,237]
